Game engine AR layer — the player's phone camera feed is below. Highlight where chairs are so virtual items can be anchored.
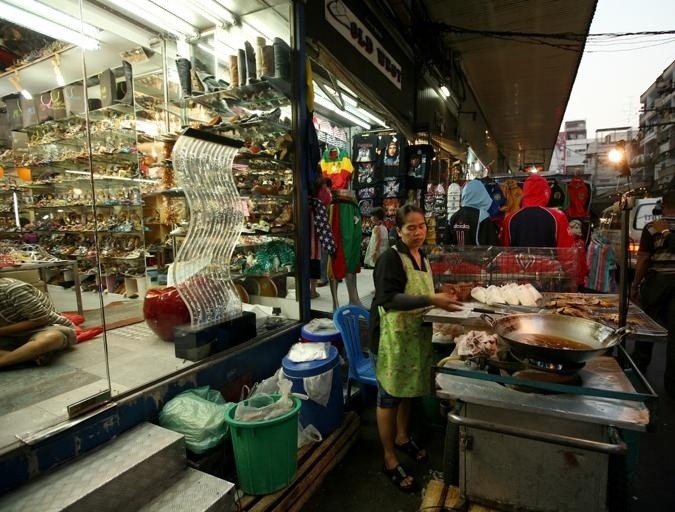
[333,304,380,412]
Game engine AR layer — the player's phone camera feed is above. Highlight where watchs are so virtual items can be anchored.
[662,228,670,234]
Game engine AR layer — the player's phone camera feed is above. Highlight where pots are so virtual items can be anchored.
[479,312,631,363]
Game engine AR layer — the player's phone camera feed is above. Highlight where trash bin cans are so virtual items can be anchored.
[282,318,345,437]
[228,397,299,495]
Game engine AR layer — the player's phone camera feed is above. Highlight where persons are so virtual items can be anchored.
[385,141,399,164]
[356,145,370,161]
[503,174,578,293]
[367,203,465,491]
[364,206,391,269]
[630,191,675,397]
[357,163,374,182]
[442,179,498,245]
[411,154,422,167]
[0,275,79,367]
[566,219,591,292]
[653,219,675,255]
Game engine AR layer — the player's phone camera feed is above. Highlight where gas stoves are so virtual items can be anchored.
[507,349,586,376]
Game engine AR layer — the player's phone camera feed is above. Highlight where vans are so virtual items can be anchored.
[590,197,663,283]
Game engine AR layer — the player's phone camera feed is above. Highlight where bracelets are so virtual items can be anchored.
[632,281,640,288]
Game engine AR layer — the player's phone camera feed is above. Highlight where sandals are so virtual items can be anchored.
[394,440,431,466]
[383,459,418,494]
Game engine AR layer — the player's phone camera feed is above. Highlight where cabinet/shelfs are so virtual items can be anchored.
[0,66,296,303]
